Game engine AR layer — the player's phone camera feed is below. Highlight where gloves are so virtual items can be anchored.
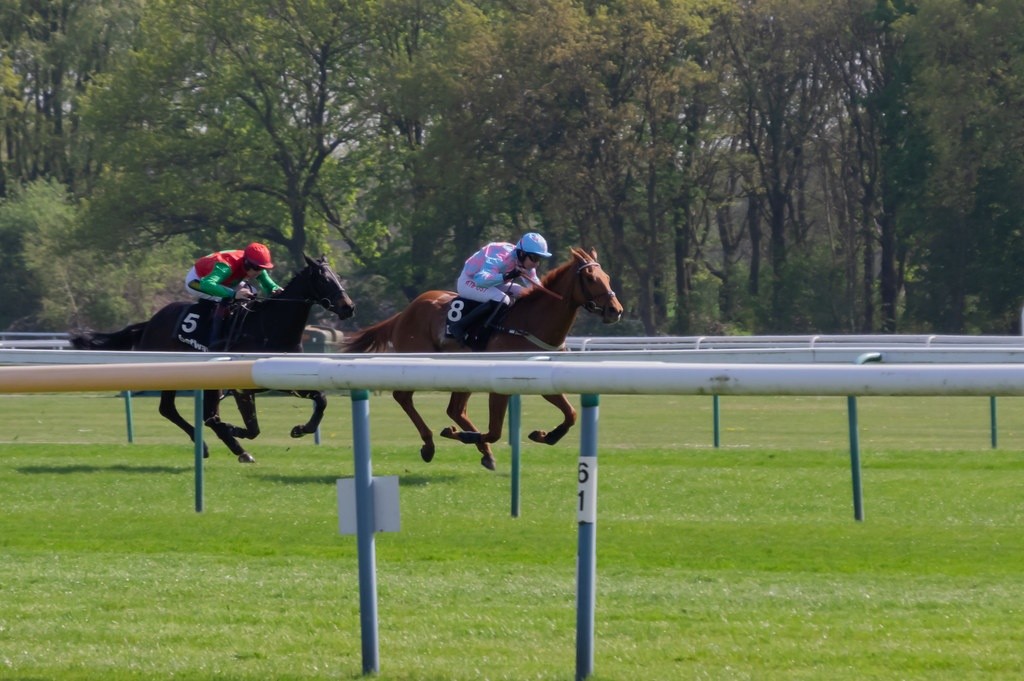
[272,287,284,294]
[503,268,522,281]
[235,288,254,301]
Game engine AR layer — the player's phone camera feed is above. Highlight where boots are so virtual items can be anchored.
[448,299,508,344]
[208,305,227,350]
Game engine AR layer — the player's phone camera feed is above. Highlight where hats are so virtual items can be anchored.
[516,232,552,257]
[245,242,274,269]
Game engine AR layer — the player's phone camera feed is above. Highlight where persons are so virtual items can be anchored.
[185,242,284,351]
[448,233,552,343]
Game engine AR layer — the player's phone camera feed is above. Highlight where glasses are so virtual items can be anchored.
[247,260,264,271]
[527,253,542,263]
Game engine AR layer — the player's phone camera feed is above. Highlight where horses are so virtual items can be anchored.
[67,250,355,464]
[336,245,625,471]
